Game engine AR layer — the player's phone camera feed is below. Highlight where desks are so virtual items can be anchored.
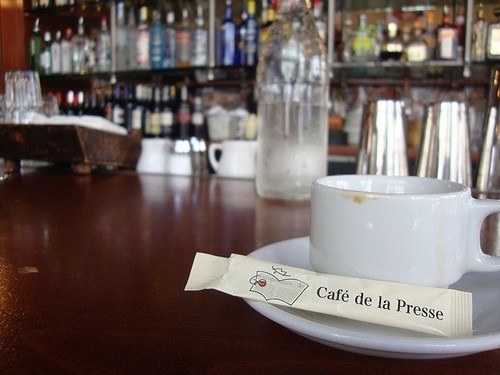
[0,169,500,375]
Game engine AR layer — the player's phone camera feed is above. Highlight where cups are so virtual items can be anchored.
[0,69,59,180]
[311,174,500,290]
[207,138,258,179]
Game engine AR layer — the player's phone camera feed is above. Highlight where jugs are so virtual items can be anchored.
[415,99,472,190]
[475,105,500,191]
[355,98,411,176]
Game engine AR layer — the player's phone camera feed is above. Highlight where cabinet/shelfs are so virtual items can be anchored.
[22,0,500,163]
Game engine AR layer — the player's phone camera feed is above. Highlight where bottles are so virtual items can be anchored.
[332,0,468,62]
[112,0,211,69]
[485,6,500,60]
[45,76,259,142]
[258,0,329,198]
[328,80,499,160]
[471,3,489,62]
[22,0,111,70]
[214,0,330,68]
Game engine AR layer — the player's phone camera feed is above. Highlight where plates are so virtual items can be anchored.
[240,235,500,358]
